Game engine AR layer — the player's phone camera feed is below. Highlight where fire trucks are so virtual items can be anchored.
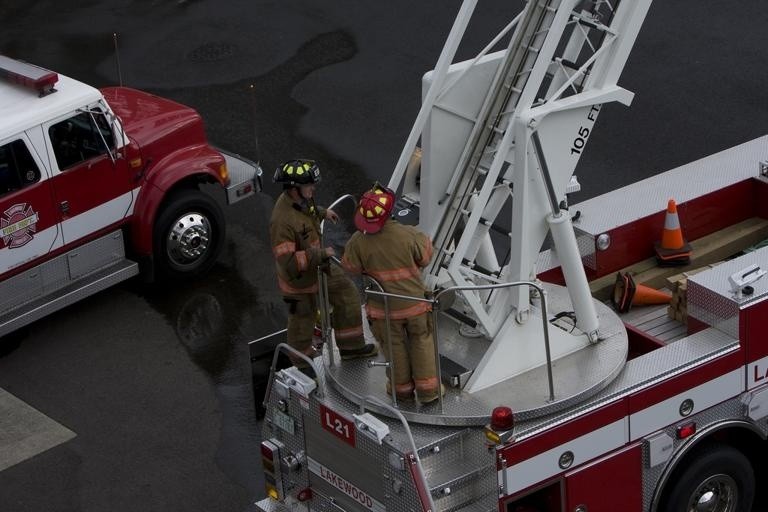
[0,56,265,341]
[253,0,766,512]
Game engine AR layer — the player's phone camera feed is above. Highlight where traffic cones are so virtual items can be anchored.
[611,272,672,312]
[652,199,694,263]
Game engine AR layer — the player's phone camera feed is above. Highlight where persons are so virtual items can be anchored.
[268,157,380,381]
[341,181,447,404]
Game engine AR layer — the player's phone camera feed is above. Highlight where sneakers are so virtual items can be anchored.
[299,366,319,387]
[339,344,378,360]
[385,381,415,403]
[418,384,446,404]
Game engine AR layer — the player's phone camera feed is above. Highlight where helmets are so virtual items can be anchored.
[272,158,322,186]
[353,186,395,234]
